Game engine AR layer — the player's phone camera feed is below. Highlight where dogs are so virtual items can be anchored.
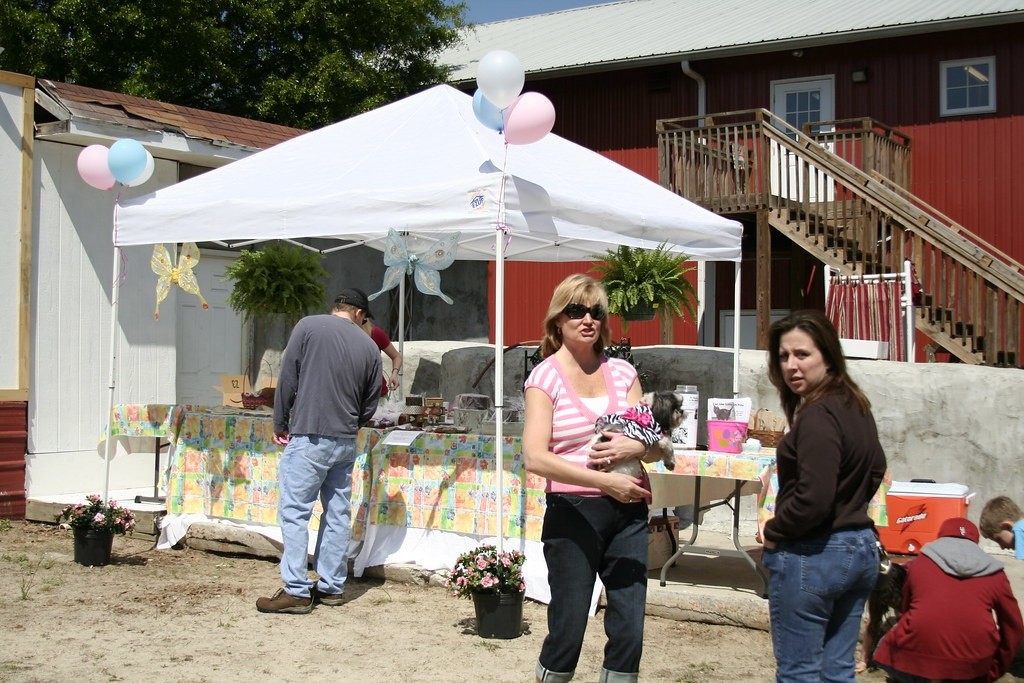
[587,392,688,480]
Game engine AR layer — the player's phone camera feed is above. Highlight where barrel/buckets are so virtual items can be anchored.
[705,419,749,454]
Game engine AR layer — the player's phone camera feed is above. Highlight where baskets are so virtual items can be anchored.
[241,358,276,409]
[747,408,785,448]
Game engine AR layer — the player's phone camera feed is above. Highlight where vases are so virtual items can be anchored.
[472,590,525,640]
[72,527,113,567]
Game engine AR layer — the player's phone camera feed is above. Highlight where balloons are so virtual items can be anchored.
[476,50,525,110]
[109,138,147,183]
[504,92,555,145]
[124,150,154,187]
[472,89,504,131]
[77,145,116,190]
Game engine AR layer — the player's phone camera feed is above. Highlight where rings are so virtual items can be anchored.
[607,457,611,465]
[629,498,632,502]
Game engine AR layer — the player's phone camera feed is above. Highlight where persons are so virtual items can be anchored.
[522,272,663,683]
[360,317,403,412]
[874,517,1024,683]
[979,496,1024,560]
[761,308,887,683]
[255,288,383,612]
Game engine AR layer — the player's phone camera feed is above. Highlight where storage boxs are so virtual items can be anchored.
[875,480,975,554]
[647,515,679,571]
[840,337,889,360]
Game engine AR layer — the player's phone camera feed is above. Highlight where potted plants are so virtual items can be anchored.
[216,238,333,327]
[585,234,700,327]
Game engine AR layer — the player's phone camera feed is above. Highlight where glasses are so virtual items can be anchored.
[562,303,605,321]
[362,309,368,325]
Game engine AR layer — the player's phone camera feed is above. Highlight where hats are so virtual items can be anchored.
[938,518,979,544]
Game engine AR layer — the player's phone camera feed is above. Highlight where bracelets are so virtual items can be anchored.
[392,368,399,374]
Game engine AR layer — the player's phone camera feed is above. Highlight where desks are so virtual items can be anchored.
[166,407,603,617]
[97,404,209,503]
[638,450,779,599]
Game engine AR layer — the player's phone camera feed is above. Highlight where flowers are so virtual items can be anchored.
[53,495,140,540]
[444,542,526,602]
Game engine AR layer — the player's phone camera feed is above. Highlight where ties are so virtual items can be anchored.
[334,288,376,321]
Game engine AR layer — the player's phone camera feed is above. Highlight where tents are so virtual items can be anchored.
[104,83,744,568]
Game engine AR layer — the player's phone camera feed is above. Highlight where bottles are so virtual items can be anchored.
[670,385,699,449]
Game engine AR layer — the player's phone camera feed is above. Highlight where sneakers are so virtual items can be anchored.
[255,588,312,614]
[310,587,343,606]
